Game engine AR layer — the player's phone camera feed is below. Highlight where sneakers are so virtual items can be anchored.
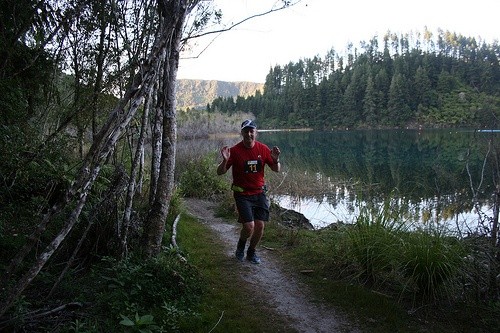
[246,254,261,263]
[236,249,244,263]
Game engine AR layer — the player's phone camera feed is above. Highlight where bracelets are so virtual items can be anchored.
[273,161,280,163]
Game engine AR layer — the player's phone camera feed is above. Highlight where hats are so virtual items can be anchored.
[241,120,257,129]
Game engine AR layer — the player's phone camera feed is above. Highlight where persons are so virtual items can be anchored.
[217,119,281,265]
